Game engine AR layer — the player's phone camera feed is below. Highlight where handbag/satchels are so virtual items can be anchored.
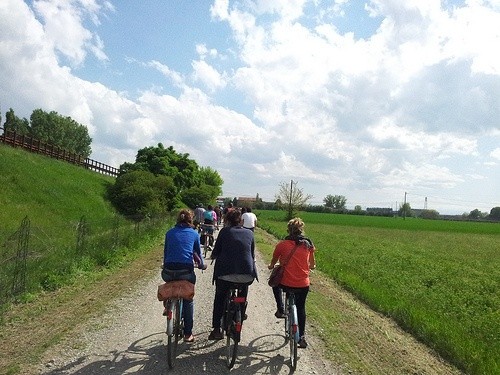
[157,280,195,302]
[267,266,284,287]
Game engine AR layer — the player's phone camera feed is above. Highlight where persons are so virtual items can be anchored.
[193,203,205,228]
[269,218,317,349]
[161,210,207,343]
[241,207,257,232]
[202,205,216,253]
[211,201,243,229]
[207,210,259,341]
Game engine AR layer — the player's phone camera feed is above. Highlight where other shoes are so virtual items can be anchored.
[298,337,307,348]
[275,308,284,318]
[163,308,173,316]
[208,245,213,251]
[242,314,247,320]
[183,334,194,342]
[208,329,222,340]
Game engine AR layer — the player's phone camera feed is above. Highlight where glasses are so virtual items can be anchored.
[287,230,290,233]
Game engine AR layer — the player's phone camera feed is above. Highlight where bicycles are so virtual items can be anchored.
[268,263,315,370]
[163,262,204,370]
[202,225,219,258]
[209,257,246,368]
